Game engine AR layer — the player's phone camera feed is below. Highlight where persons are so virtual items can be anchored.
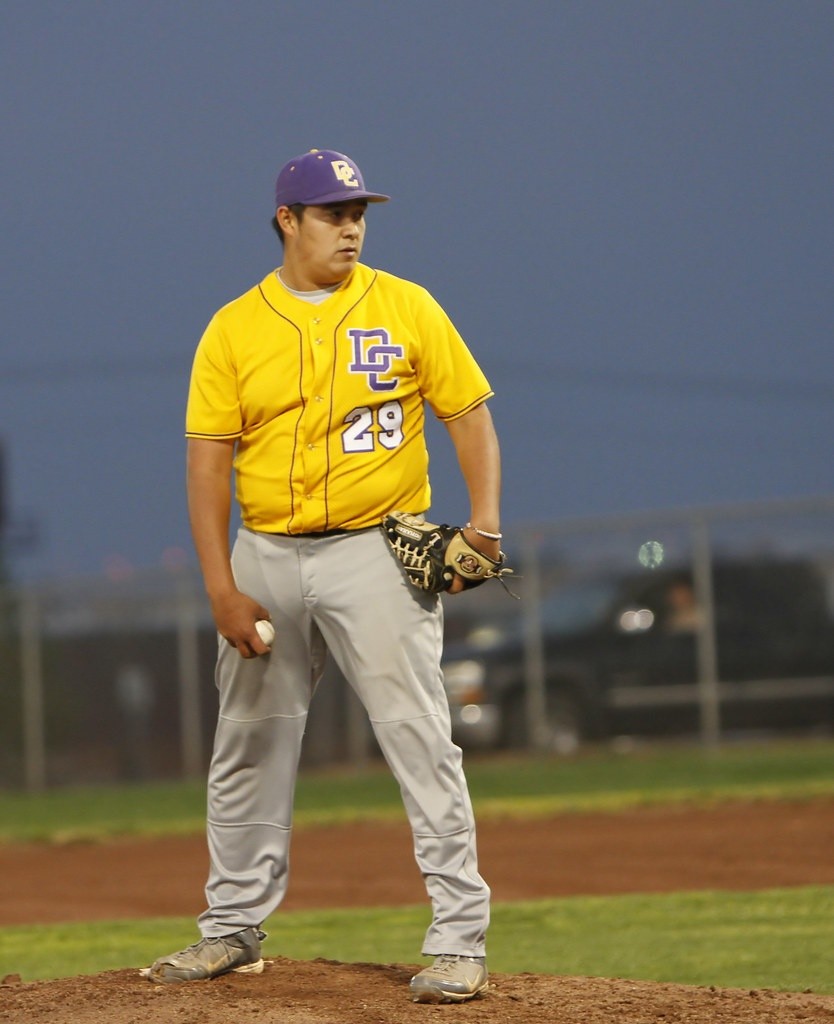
[147,145,508,1006]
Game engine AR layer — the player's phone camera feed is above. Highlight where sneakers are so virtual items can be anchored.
[411,954,489,1004]
[149,927,265,983]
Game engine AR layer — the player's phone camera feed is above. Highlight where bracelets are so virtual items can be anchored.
[466,523,503,540]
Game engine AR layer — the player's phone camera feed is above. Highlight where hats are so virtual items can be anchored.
[275,148,390,206]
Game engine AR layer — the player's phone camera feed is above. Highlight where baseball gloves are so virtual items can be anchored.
[384,511,523,601]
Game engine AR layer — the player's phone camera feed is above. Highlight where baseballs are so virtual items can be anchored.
[256,620,275,645]
